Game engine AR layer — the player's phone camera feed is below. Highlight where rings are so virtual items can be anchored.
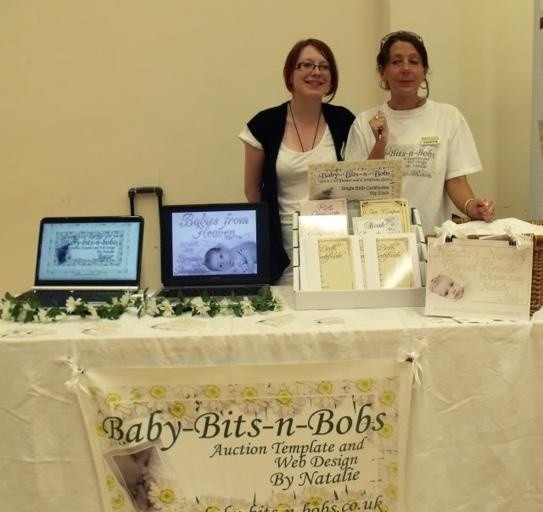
[374,115,378,119]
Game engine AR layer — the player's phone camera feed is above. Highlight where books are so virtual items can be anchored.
[296,194,423,290]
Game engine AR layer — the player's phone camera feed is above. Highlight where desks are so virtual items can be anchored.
[1,309,540,512]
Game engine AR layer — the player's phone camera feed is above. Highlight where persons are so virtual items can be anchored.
[428,274,463,301]
[343,30,495,240]
[238,40,357,288]
[202,240,257,274]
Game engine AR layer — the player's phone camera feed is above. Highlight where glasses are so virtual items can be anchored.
[381,31,423,49]
[295,63,330,71]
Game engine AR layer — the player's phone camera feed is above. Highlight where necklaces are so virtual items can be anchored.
[387,96,423,110]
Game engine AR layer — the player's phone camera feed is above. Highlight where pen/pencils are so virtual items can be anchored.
[378,130,381,140]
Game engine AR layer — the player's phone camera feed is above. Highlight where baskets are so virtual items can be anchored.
[427,218,542,314]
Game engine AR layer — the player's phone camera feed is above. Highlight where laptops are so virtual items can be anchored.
[7,215,144,308]
[147,202,271,308]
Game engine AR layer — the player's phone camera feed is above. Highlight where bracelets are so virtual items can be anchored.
[463,198,475,218]
